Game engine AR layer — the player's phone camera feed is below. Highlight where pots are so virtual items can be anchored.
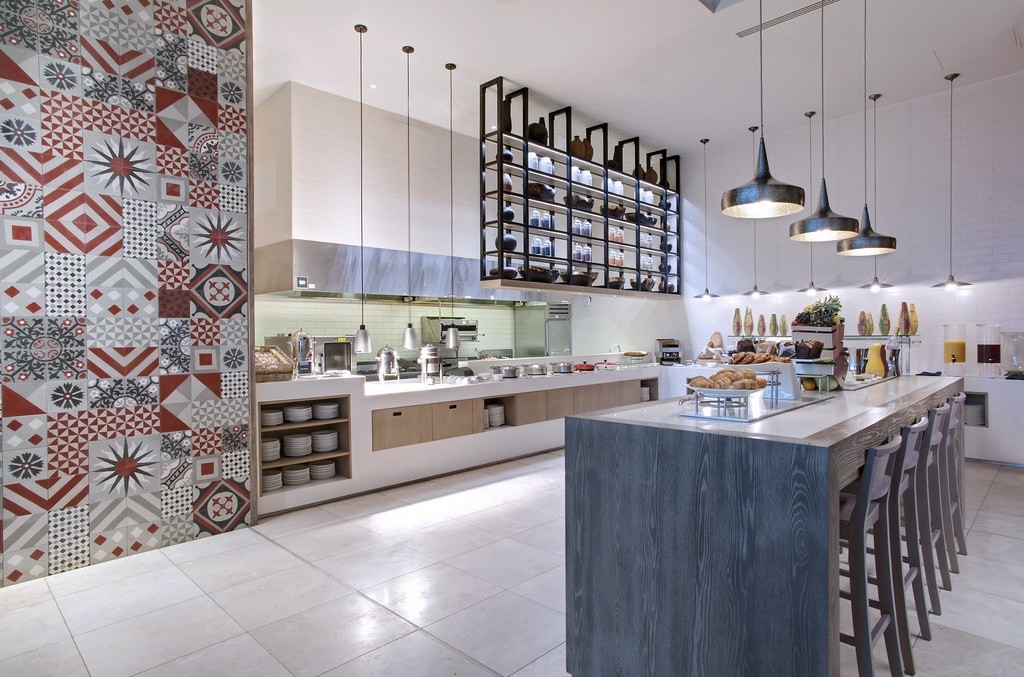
[489,359,617,378]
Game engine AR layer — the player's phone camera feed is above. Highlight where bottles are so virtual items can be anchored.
[887,326,900,348]
[880,342,889,379]
[732,306,788,336]
[864,341,886,378]
[857,301,919,336]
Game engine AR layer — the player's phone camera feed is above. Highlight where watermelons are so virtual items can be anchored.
[814,375,839,391]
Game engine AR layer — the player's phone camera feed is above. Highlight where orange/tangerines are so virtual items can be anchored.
[801,378,816,390]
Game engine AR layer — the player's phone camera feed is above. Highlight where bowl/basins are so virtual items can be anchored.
[518,265,657,291]
[491,373,504,381]
[442,375,480,385]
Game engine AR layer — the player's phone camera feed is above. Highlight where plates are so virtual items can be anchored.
[684,383,761,397]
[261,402,339,492]
[483,405,504,429]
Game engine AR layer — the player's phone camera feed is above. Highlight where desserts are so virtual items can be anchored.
[738,339,824,359]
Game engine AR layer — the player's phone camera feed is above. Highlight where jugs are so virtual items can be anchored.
[1000,331,1024,376]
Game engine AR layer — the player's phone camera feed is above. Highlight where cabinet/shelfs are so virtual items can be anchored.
[481,76,682,301]
[252,368,665,516]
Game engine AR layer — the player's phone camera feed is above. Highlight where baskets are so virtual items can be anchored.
[255,345,294,383]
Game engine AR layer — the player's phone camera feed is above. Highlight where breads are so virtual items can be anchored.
[688,367,767,390]
[728,351,790,364]
[624,351,647,355]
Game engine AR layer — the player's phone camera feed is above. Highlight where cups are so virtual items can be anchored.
[496,117,673,272]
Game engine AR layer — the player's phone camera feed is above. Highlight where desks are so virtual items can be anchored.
[564,375,964,677]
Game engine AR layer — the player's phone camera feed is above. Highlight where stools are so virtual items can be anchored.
[839,393,969,677]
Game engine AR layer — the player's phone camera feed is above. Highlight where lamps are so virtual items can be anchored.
[402,46,418,350]
[859,93,894,291]
[694,137,720,300]
[742,126,769,297]
[930,73,973,290]
[790,0,859,242]
[721,0,806,219]
[355,24,371,354]
[446,63,461,349]
[836,0,897,256]
[797,111,827,294]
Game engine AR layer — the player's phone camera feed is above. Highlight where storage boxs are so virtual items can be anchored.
[791,323,844,359]
